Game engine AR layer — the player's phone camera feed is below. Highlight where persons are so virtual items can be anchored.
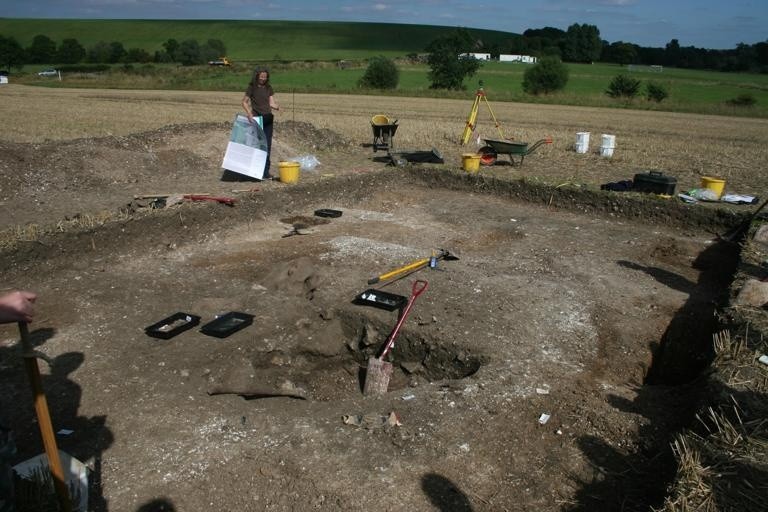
[242,66,282,181]
[0,290,37,327]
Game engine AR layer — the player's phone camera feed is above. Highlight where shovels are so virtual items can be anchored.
[363,280,427,397]
[367,248,460,285]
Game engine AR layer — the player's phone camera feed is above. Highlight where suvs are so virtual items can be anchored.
[38,69,58,77]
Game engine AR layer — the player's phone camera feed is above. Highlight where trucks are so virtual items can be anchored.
[208,58,232,67]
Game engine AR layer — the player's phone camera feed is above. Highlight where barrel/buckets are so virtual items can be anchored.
[599,146,614,156]
[461,152,482,173]
[576,144,585,153]
[371,115,390,125]
[277,162,300,185]
[602,134,615,153]
[700,176,726,200]
[576,132,590,152]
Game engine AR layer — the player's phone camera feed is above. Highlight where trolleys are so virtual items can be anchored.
[475,137,553,168]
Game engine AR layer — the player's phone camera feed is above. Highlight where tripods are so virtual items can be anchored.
[461,90,505,147]
